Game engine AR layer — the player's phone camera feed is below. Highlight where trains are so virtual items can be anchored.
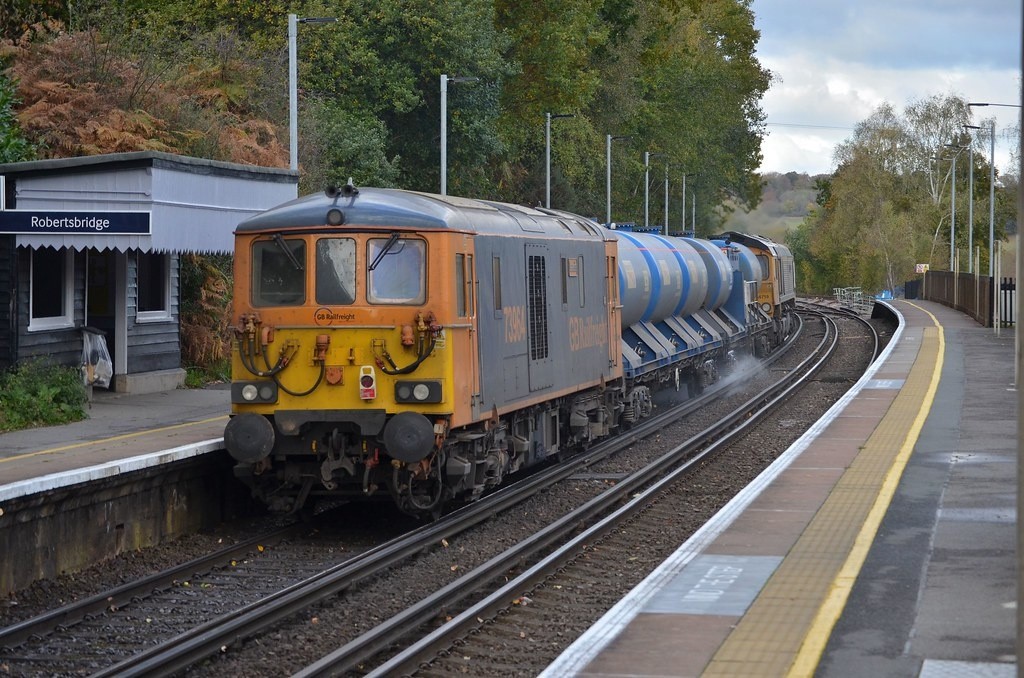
[223,176,796,528]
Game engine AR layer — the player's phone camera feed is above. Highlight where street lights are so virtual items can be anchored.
[288,14,339,170]
[930,156,955,271]
[968,102,1022,107]
[944,144,973,273]
[607,135,633,223]
[962,123,995,277]
[645,152,661,226]
[440,75,479,196]
[682,172,692,231]
[545,113,577,208]
[664,162,676,236]
